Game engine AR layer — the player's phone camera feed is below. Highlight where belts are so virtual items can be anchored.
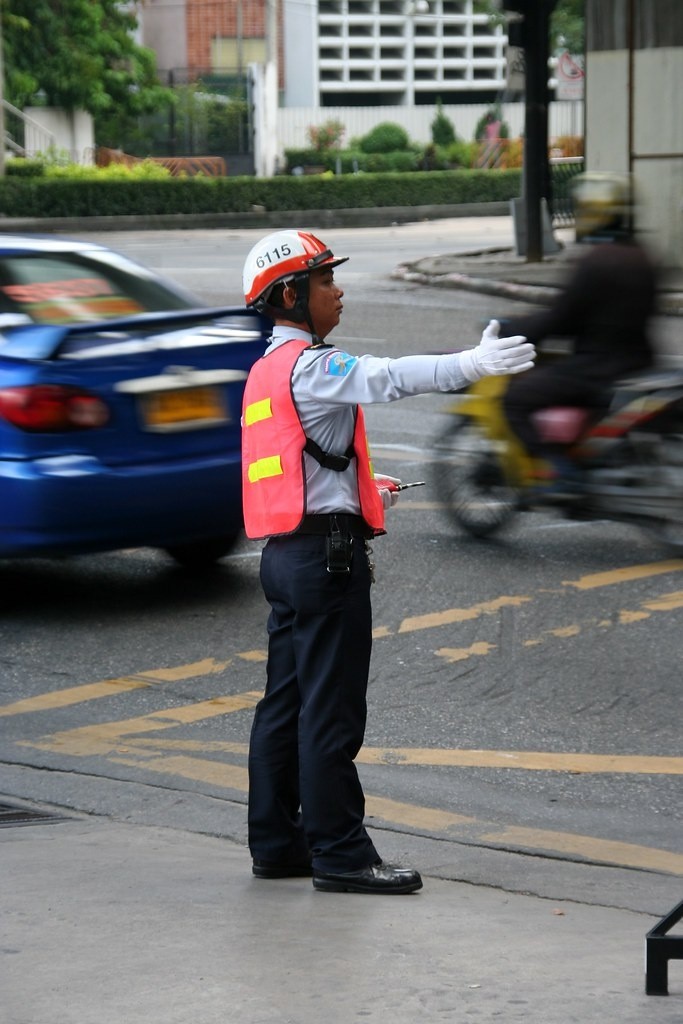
[295,514,370,534]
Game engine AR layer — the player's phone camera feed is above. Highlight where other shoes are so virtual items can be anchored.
[539,478,585,502]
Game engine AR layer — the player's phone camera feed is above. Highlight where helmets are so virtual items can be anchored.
[242,230,349,308]
[570,173,630,242]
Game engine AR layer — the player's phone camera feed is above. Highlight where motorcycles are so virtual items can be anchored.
[425,320,683,539]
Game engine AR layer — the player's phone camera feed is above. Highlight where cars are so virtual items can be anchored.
[0,237,270,557]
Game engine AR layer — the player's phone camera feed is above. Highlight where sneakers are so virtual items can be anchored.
[313,856,423,894]
[253,842,316,878]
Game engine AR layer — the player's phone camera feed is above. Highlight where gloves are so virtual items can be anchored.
[373,472,401,509]
[459,319,537,383]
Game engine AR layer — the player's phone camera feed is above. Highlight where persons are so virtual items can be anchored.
[240,231,535,894]
[485,172,654,490]
